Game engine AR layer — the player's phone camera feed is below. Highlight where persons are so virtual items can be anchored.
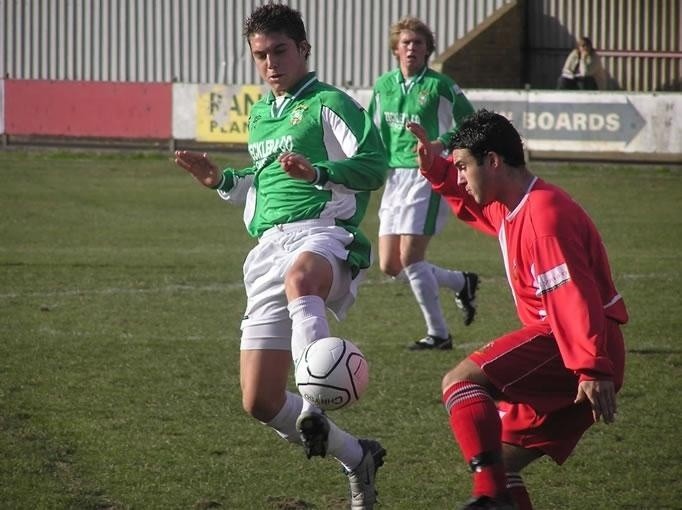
[556,37,593,88]
[172,3,389,510]
[404,108,630,510]
[365,18,481,352]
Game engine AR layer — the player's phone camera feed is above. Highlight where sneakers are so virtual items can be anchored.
[341,438,387,510]
[463,488,514,510]
[453,271,480,326]
[408,331,453,352]
[294,411,331,461]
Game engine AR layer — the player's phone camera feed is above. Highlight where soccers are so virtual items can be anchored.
[296,337,368,410]
